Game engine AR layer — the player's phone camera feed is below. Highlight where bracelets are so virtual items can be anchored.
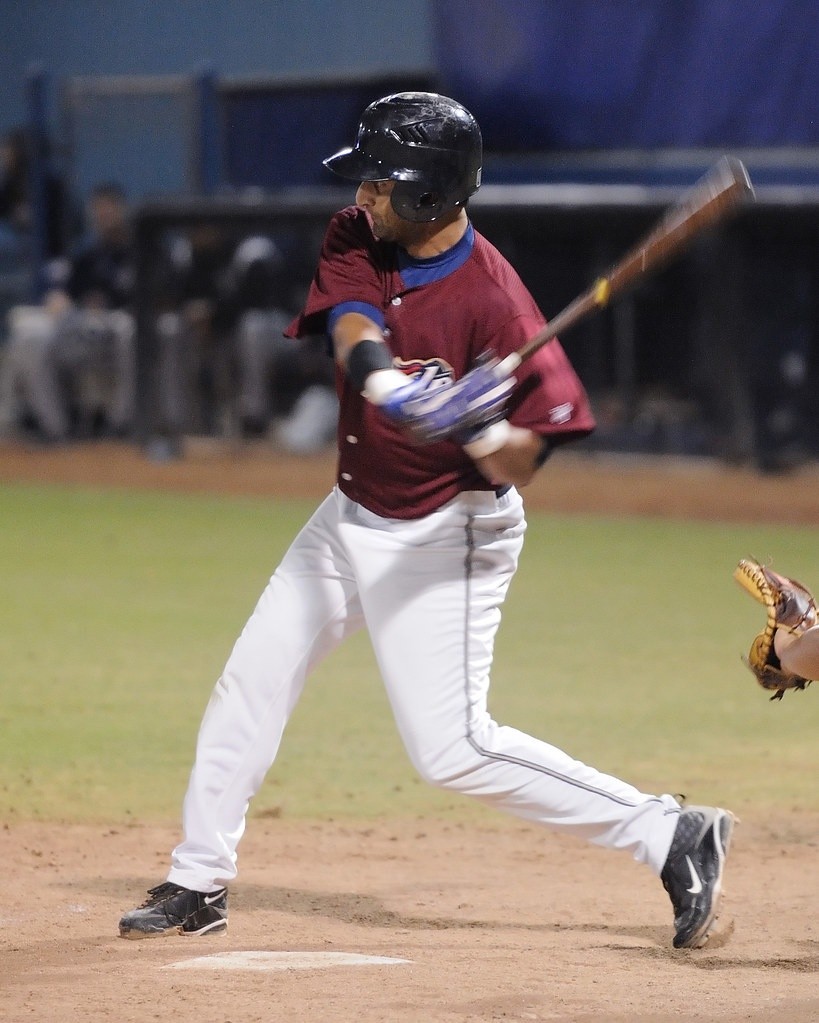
[462,418,511,462]
[347,339,392,389]
[360,370,414,407]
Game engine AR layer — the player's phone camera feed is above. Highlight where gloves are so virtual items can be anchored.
[359,354,528,459]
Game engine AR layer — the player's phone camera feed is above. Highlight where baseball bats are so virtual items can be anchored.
[495,155,758,386]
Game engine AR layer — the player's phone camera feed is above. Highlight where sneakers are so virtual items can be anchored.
[119,882,229,940]
[659,806,740,949]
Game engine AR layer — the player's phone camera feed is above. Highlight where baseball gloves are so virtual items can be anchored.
[734,558,818,692]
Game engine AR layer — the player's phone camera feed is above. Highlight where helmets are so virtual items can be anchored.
[323,92,481,221]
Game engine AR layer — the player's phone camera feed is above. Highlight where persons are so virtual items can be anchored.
[732,551,819,702]
[0,181,338,461]
[118,91,739,949]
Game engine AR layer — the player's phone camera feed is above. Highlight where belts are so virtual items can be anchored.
[496,482,515,499]
